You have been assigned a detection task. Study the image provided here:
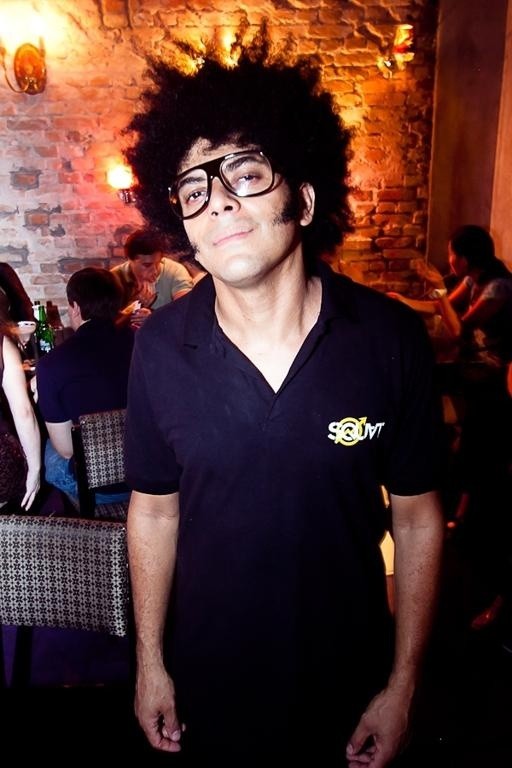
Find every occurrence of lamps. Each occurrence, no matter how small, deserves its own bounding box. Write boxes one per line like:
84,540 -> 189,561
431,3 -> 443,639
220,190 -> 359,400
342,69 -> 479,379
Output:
1,9 -> 50,96
107,165 -> 140,208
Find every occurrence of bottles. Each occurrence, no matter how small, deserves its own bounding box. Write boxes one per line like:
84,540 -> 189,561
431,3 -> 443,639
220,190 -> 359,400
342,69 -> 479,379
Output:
32,300 -> 59,356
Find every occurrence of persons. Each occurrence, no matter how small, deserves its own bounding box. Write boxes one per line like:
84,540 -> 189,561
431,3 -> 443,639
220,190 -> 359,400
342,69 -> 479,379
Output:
382,220 -> 511,368
107,228 -> 195,330
0,260 -> 36,323
29,267 -> 137,507
114,21 -> 449,767
0,328 -> 43,514
429,360 -> 511,631
384,257 -> 463,343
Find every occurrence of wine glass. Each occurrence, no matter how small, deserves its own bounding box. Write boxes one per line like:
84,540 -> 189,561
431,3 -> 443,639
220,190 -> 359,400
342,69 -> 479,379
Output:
13,325 -> 36,372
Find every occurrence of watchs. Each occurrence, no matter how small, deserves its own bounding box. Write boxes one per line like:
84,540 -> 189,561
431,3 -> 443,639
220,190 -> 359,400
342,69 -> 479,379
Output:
427,288 -> 448,301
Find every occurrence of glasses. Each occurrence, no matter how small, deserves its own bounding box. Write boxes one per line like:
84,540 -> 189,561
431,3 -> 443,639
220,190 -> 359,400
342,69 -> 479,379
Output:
159,148 -> 278,222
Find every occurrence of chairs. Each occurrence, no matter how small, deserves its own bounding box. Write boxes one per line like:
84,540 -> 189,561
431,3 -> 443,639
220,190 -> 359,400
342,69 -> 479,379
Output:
0,512 -> 131,768
72,409 -> 135,519
